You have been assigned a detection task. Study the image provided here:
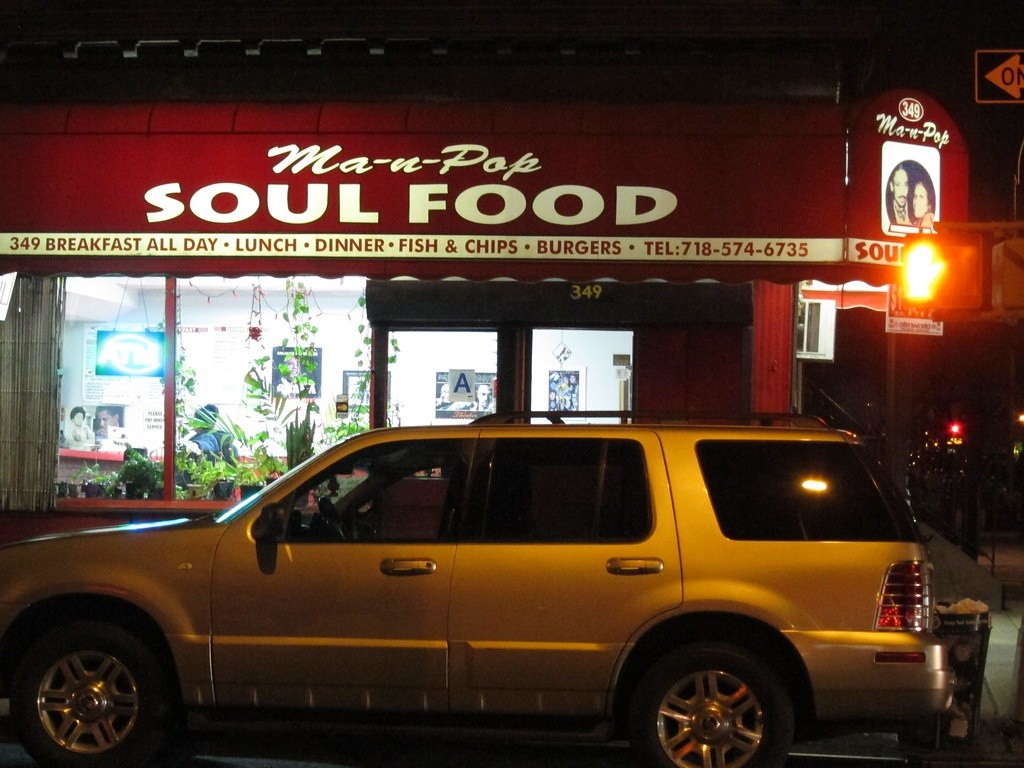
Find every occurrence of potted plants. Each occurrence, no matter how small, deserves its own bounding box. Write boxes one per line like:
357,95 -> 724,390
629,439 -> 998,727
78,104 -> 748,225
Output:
69,284 -> 401,502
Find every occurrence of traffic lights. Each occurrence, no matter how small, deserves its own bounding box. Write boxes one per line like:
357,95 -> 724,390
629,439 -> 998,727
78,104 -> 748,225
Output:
902,233 -> 986,309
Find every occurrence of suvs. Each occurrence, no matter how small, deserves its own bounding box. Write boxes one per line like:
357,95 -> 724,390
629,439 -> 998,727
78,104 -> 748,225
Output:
0,410 -> 956,768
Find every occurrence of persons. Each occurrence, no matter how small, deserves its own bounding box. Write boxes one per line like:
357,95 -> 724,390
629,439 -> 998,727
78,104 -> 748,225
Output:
64,406 -> 124,451
888,166 -> 934,227
436,382 -> 494,412
180,404 -> 240,463
278,352 -> 317,395
549,370 -> 579,411
